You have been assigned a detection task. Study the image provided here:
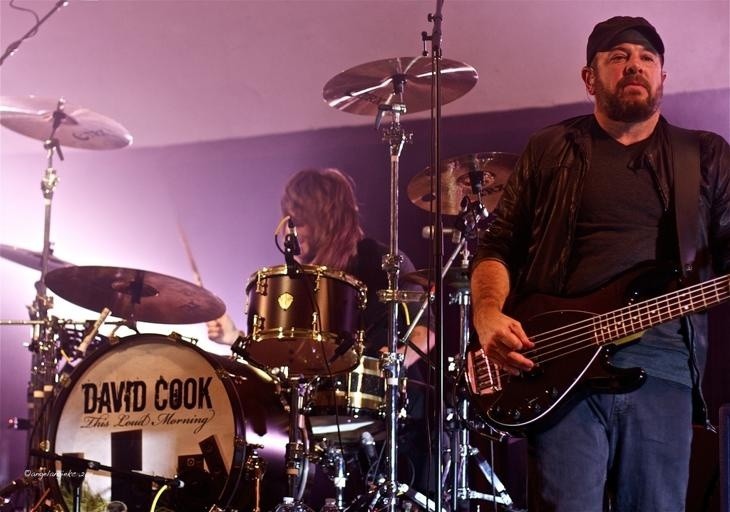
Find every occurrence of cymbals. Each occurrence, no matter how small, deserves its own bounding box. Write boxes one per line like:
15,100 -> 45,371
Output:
407,151 -> 520,215
322,56 -> 479,116
44,266 -> 226,324
0,95 -> 134,151
0,243 -> 77,273
401,264 -> 473,290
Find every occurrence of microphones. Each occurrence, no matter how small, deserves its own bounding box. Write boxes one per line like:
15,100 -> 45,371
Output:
423,226 -> 457,240
288,219 -> 301,256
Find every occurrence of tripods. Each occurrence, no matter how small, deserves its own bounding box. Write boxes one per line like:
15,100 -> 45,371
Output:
441,291 -> 513,512
341,104 -> 447,512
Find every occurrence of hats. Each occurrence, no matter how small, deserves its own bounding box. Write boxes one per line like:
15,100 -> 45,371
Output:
586,17 -> 665,67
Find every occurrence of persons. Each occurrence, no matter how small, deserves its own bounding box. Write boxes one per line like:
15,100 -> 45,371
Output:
467,14 -> 729,511
203,164 -> 438,369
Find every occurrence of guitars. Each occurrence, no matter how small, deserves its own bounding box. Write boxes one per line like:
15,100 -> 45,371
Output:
467,273 -> 730,439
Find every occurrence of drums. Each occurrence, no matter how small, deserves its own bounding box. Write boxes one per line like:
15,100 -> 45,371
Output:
245,263 -> 368,377
44,333 -> 316,512
309,348 -> 411,446
